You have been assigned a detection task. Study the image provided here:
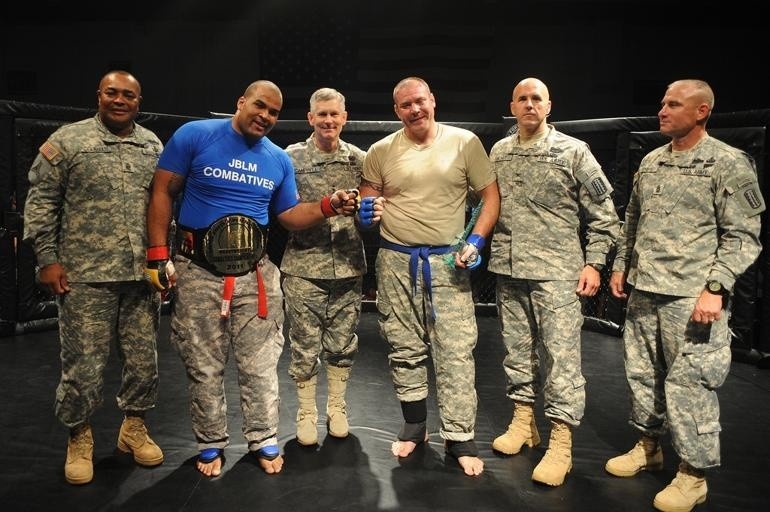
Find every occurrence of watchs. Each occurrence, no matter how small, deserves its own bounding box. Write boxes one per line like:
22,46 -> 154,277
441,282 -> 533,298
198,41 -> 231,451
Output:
704,280 -> 730,299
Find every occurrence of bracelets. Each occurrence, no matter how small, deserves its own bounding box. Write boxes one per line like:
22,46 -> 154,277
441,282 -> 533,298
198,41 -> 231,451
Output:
589,262 -> 604,272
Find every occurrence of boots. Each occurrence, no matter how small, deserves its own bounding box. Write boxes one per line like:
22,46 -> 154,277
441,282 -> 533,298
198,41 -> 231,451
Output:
654,464 -> 707,512
117,411 -> 164,467
604,437 -> 664,476
296,375 -> 318,447
64,423 -> 94,484
493,400 -> 541,457
530,418 -> 572,488
326,366 -> 349,439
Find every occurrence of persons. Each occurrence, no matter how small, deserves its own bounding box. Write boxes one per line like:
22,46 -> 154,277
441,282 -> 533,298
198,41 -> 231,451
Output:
605,79 -> 766,512
354,74 -> 502,475
277,86 -> 369,449
146,79 -> 361,477
24,70 -> 167,485
489,76 -> 620,486
